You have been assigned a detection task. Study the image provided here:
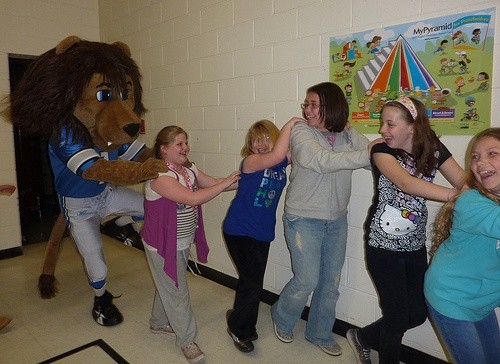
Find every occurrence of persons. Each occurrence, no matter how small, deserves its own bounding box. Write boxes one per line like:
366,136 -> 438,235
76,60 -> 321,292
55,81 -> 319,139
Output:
346,97 -> 470,364
0,184 -> 16,331
270,82 -> 387,356
422,127 -> 500,364
222,117 -> 351,353
139,125 -> 241,364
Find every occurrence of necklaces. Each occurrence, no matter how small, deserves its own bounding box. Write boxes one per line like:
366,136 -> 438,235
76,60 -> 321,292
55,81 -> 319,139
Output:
167,165 -> 194,192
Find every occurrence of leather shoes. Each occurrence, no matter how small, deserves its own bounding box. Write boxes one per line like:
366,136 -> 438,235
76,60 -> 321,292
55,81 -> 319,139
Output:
226,309 -> 258,352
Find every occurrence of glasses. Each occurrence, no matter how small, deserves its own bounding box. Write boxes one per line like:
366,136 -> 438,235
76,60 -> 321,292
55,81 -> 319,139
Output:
253,136 -> 270,143
301,103 -> 321,109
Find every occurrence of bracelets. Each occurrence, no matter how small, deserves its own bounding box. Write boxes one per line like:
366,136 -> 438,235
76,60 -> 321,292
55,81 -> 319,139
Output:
447,188 -> 455,202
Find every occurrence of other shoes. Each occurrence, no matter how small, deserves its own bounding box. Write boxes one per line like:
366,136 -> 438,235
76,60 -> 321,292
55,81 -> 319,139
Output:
0,316 -> 13,330
108,217 -> 141,245
91,292 -> 123,327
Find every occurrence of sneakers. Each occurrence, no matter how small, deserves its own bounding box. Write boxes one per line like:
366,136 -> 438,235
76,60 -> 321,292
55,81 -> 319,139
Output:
305,331 -> 342,356
150,323 -> 176,336
180,342 -> 205,363
273,320 -> 294,343
346,328 -> 372,364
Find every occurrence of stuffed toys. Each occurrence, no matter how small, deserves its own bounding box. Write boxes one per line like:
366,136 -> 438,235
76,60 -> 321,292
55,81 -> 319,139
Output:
0,35 -> 192,327
